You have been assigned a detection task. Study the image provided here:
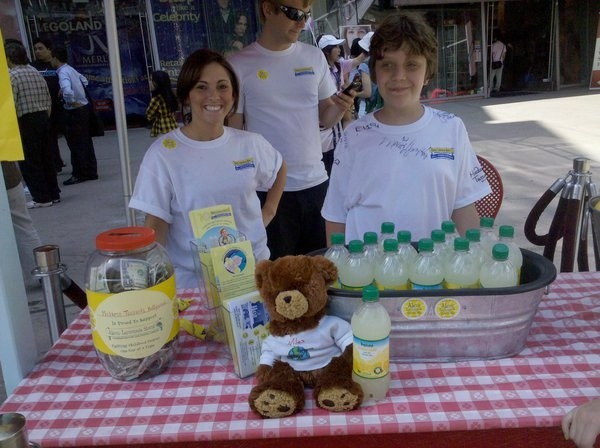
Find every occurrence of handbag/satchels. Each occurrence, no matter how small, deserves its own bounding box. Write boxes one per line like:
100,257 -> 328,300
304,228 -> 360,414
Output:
491,43 -> 504,70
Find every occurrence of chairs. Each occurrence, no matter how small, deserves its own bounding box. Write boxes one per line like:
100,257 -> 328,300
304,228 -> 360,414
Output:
472,155 -> 504,221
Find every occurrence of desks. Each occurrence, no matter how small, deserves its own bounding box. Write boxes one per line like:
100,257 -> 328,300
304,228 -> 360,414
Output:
0,270 -> 600,447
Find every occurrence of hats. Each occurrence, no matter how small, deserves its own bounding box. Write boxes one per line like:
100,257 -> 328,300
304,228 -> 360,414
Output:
357,31 -> 375,53
318,34 -> 346,50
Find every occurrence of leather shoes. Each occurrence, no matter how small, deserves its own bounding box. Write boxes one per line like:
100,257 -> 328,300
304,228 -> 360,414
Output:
81,175 -> 98,180
63,176 -> 85,185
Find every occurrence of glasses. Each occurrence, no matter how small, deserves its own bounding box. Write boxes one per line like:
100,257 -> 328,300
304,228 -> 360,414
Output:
279,3 -> 312,22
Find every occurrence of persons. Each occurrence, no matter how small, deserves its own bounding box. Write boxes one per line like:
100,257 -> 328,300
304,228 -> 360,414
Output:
318,119 -> 342,177
146,71 -> 180,138
50,47 -> 98,186
29,42 -> 68,175
0,28 -> 43,288
321,13 -> 492,248
467,32 -> 490,96
350,37 -> 363,84
224,9 -> 246,51
313,34 -> 369,95
562,397 -> 600,448
347,31 -> 384,119
2,38 -> 60,208
222,0 -> 361,262
216,0 -> 234,34
486,36 -> 507,92
128,49 -> 287,290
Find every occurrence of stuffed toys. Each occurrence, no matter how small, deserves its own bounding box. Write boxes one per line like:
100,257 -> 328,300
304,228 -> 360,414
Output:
249,253 -> 364,420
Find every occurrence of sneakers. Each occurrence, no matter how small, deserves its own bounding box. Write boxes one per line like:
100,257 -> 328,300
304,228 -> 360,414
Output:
26,200 -> 52,208
52,198 -> 60,202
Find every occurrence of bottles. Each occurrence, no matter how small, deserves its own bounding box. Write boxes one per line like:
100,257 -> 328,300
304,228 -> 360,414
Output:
446,237 -> 479,289
431,229 -> 448,260
350,285 -> 391,402
324,233 -> 349,290
442,221 -> 459,249
497,225 -> 523,284
364,232 -> 381,262
480,244 -> 517,288
83,226 -> 180,382
465,229 -> 487,261
397,230 -> 419,263
479,218 -> 499,248
409,238 -> 444,290
375,239 -> 409,290
378,222 -> 399,246
339,240 -> 375,291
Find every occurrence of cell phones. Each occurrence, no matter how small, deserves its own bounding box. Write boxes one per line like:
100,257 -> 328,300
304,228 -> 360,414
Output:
340,72 -> 362,96
